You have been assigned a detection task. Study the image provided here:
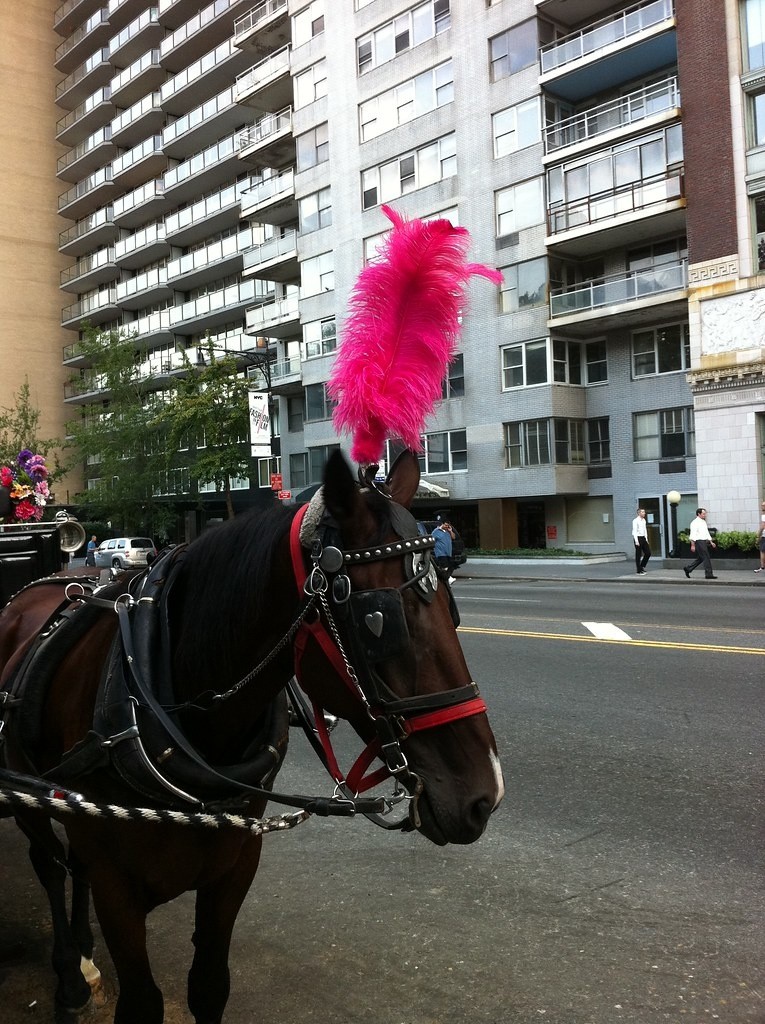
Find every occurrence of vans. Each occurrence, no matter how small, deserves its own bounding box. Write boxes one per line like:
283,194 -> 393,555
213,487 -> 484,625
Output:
84,537 -> 158,570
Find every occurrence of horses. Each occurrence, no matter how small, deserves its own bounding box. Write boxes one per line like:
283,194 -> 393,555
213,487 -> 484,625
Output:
0,443 -> 504,1024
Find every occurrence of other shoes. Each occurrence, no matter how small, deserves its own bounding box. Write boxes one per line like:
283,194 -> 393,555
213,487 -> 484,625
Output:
705,575 -> 717,579
641,566 -> 648,574
683,567 -> 690,578
448,576 -> 456,585
636,571 -> 645,575
754,568 -> 765,573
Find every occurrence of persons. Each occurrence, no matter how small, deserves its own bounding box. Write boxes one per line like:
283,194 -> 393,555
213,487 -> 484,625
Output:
754,502 -> 765,573
632,508 -> 651,575
87,535 -> 99,567
682,508 -> 718,579
431,518 -> 457,585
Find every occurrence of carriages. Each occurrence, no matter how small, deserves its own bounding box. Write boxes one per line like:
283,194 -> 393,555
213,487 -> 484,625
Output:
0,444 -> 506,1024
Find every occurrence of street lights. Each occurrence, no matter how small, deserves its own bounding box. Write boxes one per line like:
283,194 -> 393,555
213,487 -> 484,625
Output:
666,490 -> 682,558
194,346 -> 277,474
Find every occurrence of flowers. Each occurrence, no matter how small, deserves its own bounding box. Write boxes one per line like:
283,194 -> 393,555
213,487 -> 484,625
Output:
0,445 -> 52,522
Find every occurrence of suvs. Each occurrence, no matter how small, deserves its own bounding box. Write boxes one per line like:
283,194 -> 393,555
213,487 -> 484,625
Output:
415,519 -> 467,570
